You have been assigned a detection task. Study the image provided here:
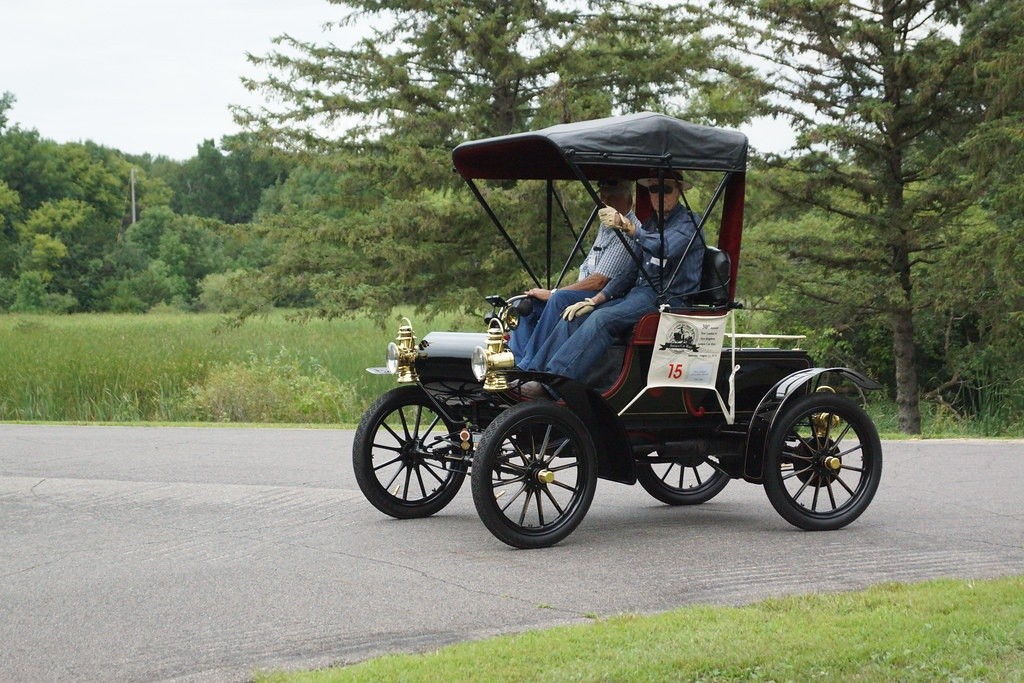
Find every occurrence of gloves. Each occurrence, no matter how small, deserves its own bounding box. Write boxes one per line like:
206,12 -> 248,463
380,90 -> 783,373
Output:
559,297 -> 596,321
597,201 -> 635,236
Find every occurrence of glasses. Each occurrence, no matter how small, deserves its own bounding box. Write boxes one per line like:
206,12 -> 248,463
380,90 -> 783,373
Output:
649,184 -> 679,194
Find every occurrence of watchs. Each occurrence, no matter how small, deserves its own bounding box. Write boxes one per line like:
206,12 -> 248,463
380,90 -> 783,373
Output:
623,221 -> 632,232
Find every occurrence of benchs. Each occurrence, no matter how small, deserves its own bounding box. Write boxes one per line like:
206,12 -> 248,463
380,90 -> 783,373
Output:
591,246 -> 731,398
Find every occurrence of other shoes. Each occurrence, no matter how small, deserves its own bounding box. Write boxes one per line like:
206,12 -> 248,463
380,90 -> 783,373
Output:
508,378 -> 558,403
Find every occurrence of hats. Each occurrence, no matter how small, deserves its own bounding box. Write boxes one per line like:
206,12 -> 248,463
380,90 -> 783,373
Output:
595,177 -> 635,193
637,168 -> 693,190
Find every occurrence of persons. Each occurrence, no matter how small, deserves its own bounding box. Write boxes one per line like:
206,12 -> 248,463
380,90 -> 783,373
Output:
508,169 -> 706,402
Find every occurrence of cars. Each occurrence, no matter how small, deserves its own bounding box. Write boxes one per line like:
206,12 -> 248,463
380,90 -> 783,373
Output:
351,111 -> 883,552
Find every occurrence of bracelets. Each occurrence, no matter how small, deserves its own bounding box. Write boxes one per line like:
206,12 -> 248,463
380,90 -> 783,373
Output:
551,289 -> 557,295
